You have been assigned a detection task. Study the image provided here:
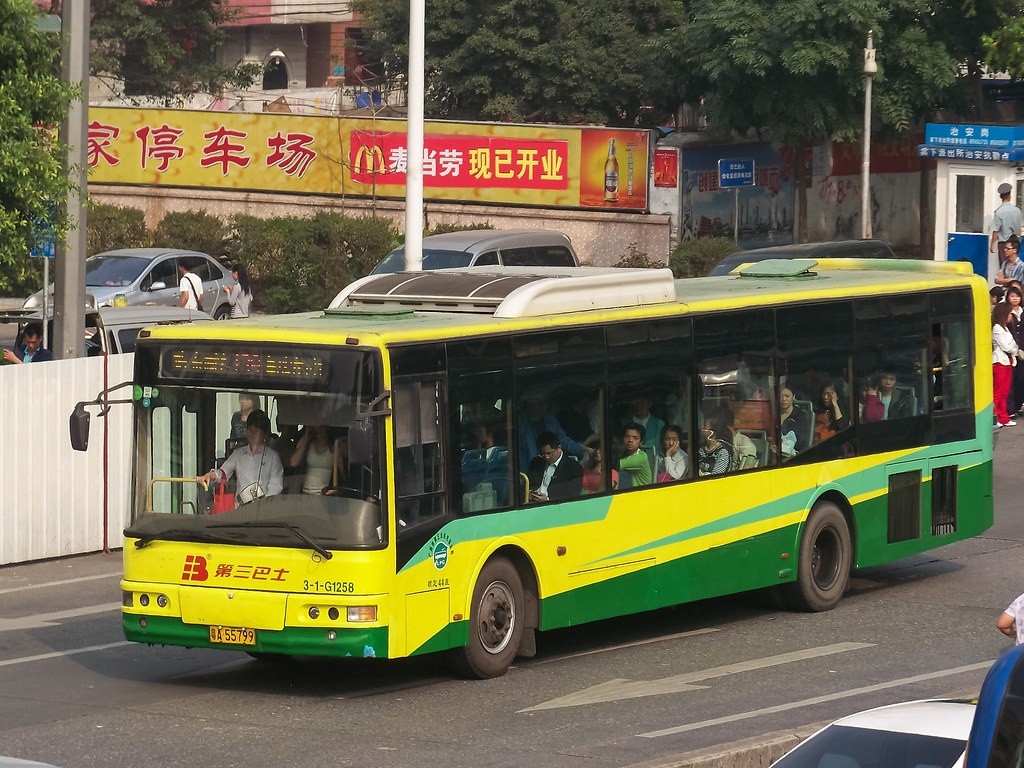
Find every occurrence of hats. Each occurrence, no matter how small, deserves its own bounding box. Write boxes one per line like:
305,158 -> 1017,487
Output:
997,183 -> 1012,194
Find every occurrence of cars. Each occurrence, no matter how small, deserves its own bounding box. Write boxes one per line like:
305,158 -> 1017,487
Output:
21,245 -> 237,318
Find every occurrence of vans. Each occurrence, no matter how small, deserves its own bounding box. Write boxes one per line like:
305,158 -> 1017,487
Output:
703,239 -> 901,276
369,227 -> 581,275
0,308 -> 218,354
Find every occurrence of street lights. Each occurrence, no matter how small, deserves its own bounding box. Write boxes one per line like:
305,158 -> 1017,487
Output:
864,50 -> 877,240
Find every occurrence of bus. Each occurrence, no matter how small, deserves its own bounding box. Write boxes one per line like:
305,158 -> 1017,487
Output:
69,255 -> 995,679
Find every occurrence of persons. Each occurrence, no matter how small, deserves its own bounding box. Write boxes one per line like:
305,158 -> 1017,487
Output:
996,593 -> 1024,646
193,334 -> 948,509
987,184 -> 1024,428
175,259 -> 204,312
2,323 -> 52,364
224,263 -> 253,320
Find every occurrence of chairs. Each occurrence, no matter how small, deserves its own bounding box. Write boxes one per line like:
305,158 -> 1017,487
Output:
793,400 -> 815,454
895,385 -> 917,418
735,429 -> 769,470
817,737 -> 866,768
638,444 -> 659,485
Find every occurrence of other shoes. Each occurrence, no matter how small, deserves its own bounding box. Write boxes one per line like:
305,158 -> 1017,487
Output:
997,422 -> 1003,428
1004,420 -> 1017,426
1009,414 -> 1017,420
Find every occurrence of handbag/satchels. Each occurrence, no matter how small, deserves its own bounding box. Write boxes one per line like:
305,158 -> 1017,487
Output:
197,300 -> 203,311
211,469 -> 236,513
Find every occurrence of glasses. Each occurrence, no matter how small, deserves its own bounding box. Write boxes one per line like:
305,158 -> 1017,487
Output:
538,448 -> 556,460
1004,247 -> 1014,251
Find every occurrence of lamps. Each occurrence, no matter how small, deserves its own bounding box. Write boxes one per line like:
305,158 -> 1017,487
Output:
269,47 -> 286,65
